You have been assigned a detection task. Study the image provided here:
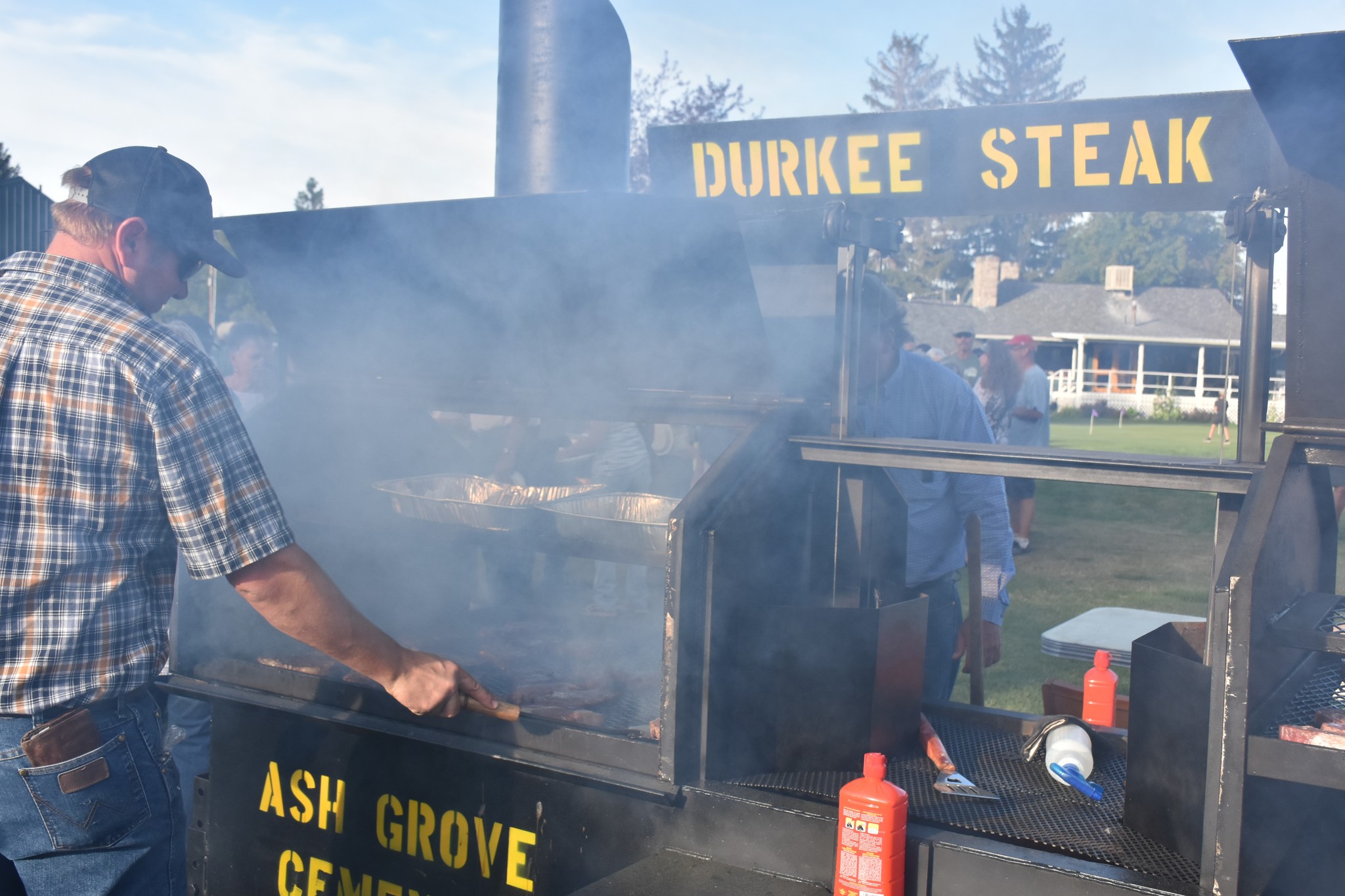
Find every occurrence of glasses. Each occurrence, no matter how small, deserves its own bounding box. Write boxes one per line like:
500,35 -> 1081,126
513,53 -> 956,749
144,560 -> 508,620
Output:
955,332 -> 972,338
166,238 -> 207,283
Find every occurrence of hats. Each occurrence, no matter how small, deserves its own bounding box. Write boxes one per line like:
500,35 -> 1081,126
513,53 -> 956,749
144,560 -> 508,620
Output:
926,347 -> 946,362
952,322 -> 976,336
68,145 -> 250,277
1005,333 -> 1037,352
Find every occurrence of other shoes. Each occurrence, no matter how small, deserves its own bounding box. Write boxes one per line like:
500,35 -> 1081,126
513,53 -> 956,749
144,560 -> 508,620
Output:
1011,540 -> 1031,556
1224,441 -> 1230,445
1203,439 -> 1210,443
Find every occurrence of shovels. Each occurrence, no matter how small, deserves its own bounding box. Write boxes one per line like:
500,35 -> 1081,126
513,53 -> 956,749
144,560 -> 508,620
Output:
919,712 -> 1000,799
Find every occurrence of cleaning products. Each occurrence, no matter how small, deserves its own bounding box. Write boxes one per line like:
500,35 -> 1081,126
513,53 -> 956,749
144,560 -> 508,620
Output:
1041,723 -> 1107,803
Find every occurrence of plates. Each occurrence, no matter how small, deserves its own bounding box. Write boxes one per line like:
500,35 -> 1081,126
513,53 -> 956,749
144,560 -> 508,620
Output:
1039,607 -> 1206,668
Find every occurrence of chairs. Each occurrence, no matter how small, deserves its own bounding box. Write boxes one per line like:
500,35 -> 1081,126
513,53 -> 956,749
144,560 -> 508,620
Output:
1042,677 -> 1129,730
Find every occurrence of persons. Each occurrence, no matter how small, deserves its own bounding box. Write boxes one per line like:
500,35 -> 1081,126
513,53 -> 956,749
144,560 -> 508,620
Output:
159,313 -> 244,418
1004,330 -> 1049,552
0,146 -> 501,895
904,336 -> 945,361
839,276 -> 1016,701
223,321 -> 271,411
938,323 -> 980,388
972,339 -> 1022,446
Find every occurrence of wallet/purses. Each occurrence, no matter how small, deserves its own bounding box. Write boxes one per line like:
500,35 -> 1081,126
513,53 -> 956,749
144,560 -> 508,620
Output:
21,704 -> 101,772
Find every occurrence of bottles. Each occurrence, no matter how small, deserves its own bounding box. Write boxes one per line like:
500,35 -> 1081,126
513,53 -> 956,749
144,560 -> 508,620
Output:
833,752 -> 908,896
1081,649 -> 1118,728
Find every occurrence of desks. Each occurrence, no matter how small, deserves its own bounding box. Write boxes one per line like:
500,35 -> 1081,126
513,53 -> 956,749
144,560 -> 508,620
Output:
1041,607 -> 1207,667
